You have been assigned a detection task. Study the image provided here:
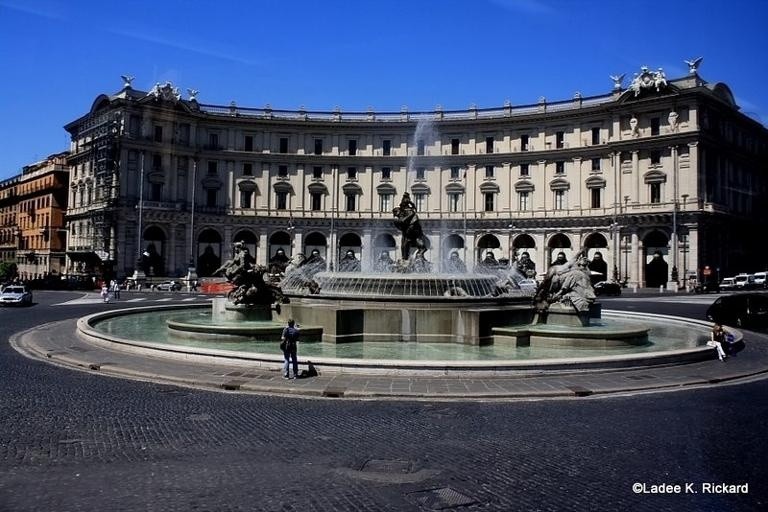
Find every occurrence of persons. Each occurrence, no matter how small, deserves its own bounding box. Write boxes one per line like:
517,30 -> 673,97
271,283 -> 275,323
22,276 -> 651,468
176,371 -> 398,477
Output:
549,269 -> 596,313
391,206 -> 430,262
705,323 -> 727,364
281,319 -> 299,380
226,240 -> 250,276
649,68 -> 667,92
91,275 -> 136,304
631,72 -> 645,97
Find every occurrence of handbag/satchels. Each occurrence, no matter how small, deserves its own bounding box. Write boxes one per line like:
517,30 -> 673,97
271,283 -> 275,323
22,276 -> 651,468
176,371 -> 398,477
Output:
280,341 -> 288,351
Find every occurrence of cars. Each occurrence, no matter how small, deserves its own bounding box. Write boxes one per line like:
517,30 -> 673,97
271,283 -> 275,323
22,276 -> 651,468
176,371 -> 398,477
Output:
157,280 -> 182,291
706,293 -> 767,333
719,271 -> 768,289
518,278 -> 539,290
0,285 -> 33,306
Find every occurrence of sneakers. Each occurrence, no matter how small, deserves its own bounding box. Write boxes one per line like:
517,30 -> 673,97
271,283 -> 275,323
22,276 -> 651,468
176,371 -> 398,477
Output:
282,374 -> 296,380
